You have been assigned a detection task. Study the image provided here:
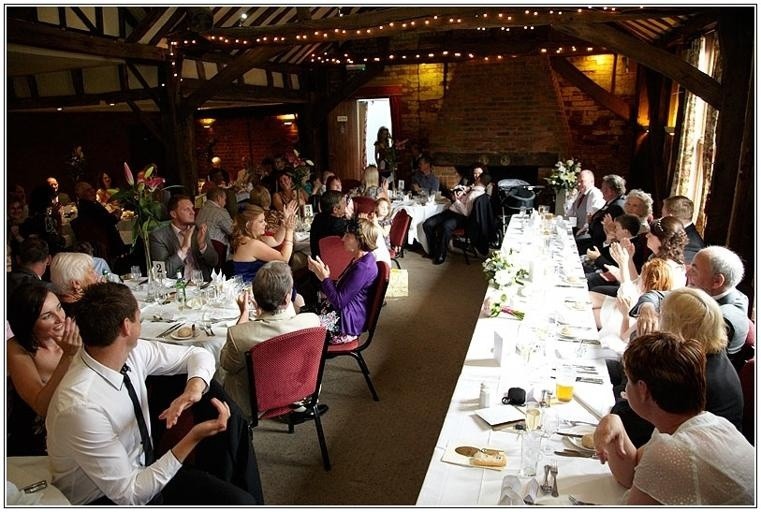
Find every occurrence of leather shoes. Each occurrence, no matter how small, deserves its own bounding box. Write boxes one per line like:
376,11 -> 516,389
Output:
432,253 -> 447,264
292,403 -> 329,424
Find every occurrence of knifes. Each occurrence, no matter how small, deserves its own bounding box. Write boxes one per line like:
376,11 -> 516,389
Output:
154,321 -> 179,337
160,323 -> 185,338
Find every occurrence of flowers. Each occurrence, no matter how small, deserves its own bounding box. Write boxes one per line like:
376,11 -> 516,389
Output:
105,161 -> 186,273
538,156 -> 587,193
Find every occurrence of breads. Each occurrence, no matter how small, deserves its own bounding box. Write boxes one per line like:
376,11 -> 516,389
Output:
582,433 -> 595,448
177,327 -> 191,337
475,451 -> 505,466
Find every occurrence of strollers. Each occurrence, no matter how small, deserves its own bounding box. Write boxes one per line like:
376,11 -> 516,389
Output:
493,178 -> 547,249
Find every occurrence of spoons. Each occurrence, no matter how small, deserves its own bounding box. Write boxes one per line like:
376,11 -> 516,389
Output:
205,323 -> 216,338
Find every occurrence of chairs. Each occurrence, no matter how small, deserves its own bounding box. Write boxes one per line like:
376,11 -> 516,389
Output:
4,174 -> 493,303
324,261 -> 390,402
247,327 -> 332,471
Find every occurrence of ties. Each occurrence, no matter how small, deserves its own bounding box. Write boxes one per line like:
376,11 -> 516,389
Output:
121,372 -> 154,466
179,233 -> 194,266
577,194 -> 586,207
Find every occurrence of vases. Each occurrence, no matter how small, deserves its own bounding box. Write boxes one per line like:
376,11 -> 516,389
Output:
550,187 -> 581,218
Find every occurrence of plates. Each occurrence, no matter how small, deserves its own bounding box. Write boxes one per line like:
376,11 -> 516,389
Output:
169,329 -> 192,340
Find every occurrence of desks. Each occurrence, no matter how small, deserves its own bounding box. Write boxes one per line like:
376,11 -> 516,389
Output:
7,463 -> 71,508
413,213 -> 636,505
118,277 -> 258,388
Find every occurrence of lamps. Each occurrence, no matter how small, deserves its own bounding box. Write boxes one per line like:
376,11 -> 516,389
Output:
198,118 -> 216,129
276,113 -> 297,127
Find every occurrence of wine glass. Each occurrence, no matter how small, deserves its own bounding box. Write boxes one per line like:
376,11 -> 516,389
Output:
94,258 -> 257,325
388,185 -> 449,208
292,215 -> 310,239
467,204 -> 617,505
